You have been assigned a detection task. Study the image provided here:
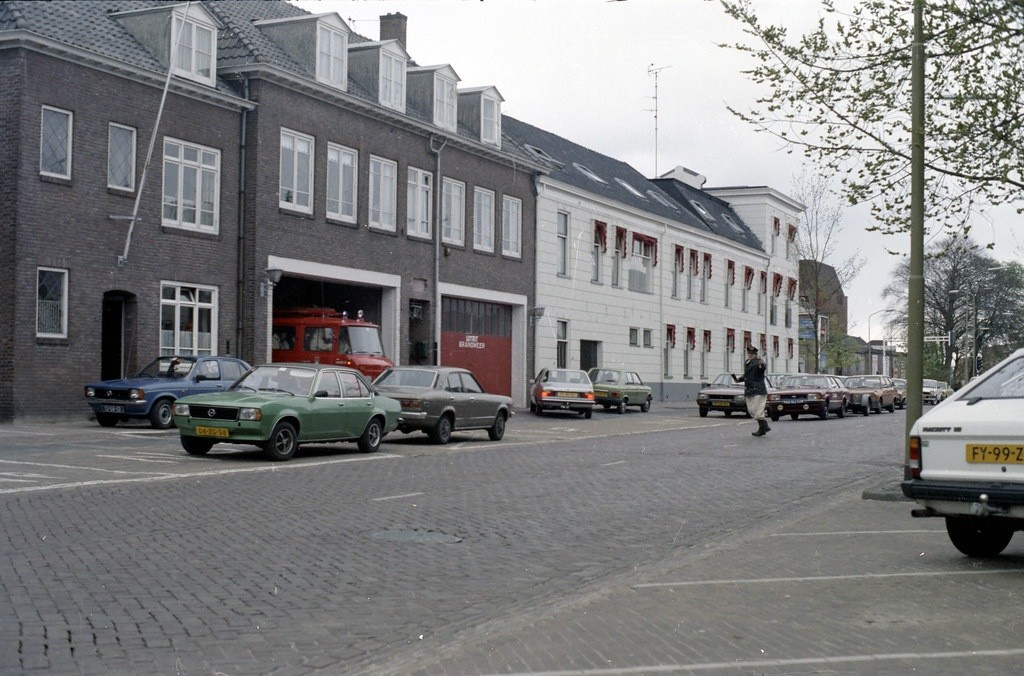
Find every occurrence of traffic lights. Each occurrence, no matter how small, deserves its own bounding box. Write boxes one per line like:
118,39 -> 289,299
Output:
977,356 -> 983,370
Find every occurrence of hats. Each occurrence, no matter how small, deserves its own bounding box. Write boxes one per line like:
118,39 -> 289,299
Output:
746,347 -> 758,355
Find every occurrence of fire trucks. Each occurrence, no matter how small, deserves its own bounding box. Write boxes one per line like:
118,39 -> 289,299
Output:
270,305 -> 396,386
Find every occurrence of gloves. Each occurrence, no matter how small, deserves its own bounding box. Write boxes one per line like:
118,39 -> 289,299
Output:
738,376 -> 744,382
757,363 -> 765,369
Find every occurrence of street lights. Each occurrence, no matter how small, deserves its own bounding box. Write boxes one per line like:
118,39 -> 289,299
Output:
948,289 -> 969,385
869,308 -> 896,374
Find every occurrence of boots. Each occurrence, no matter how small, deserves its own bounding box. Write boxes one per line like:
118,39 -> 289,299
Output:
751,419 -> 771,436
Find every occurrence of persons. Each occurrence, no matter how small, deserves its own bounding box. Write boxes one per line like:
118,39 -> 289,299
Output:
731,347 -> 772,438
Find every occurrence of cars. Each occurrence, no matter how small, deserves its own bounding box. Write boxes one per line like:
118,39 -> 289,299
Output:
765,374 -> 851,421
843,375 -> 898,417
939,381 -> 956,402
172,363 -> 401,463
919,379 -> 942,406
901,347 -> 1024,557
371,365 -> 515,445
889,378 -> 910,410
697,371 -> 773,418
83,354 -> 252,431
587,367 -> 655,414
528,368 -> 596,419
